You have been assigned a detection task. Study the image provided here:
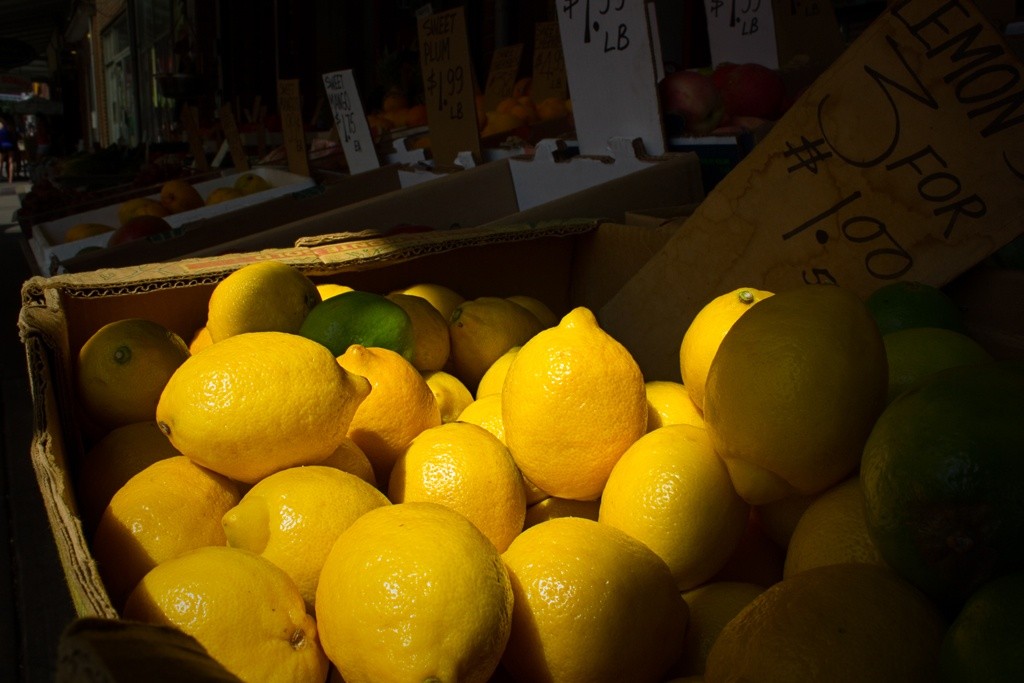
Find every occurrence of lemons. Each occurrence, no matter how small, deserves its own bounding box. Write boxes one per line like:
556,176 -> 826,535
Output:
365,97 -> 571,149
71,260 -> 1024,683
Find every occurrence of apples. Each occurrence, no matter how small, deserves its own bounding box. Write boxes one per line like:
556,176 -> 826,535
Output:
660,62 -> 789,138
66,174 -> 270,242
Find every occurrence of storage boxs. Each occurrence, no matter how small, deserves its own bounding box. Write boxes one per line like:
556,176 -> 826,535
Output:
17,58 -> 1024,683
